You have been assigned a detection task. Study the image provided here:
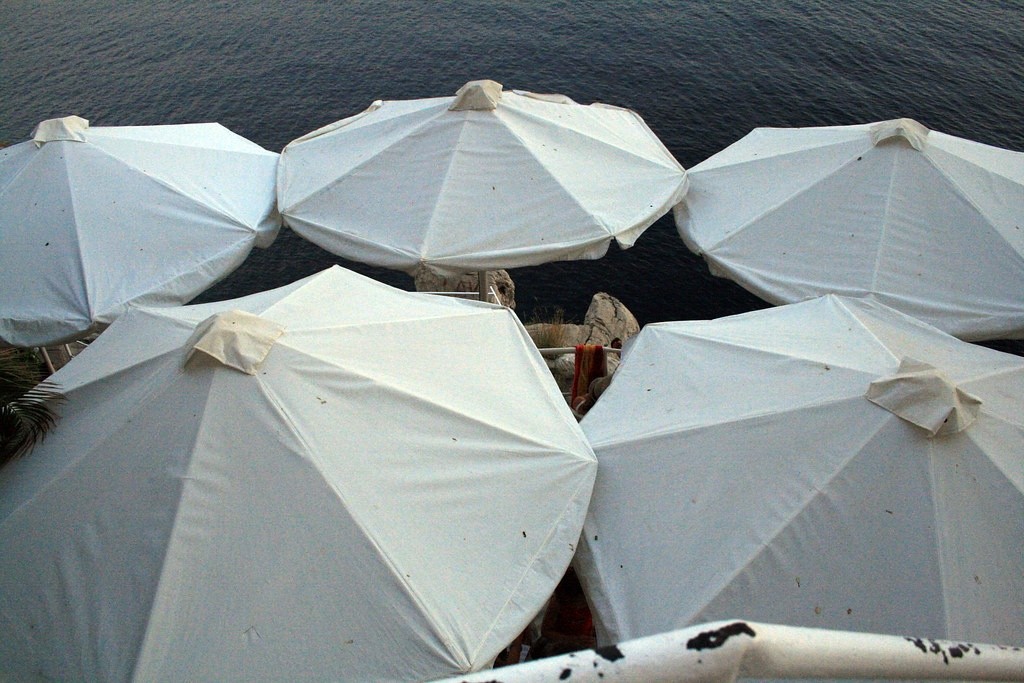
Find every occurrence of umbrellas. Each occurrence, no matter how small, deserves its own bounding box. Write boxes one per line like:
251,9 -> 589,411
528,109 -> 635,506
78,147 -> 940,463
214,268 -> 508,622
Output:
0,81 -> 1024,683
